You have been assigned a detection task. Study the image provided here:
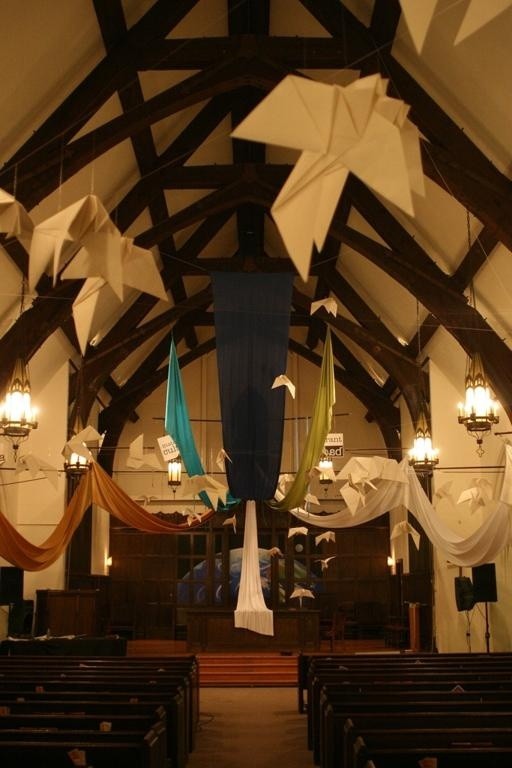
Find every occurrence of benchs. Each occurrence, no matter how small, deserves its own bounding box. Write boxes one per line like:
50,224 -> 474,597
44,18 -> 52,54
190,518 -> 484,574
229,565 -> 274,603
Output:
1,654 -> 201,767
297,653 -> 511,766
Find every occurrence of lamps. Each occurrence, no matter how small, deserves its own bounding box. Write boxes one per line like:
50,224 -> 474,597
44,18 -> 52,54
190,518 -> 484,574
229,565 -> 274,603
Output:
405,137 -> 502,476
62,357 -> 93,479
0,261 -> 39,462
319,452 -> 338,495
167,454 -> 183,494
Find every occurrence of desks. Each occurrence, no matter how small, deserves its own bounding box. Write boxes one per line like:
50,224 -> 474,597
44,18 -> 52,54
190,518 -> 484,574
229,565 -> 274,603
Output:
1,635 -> 127,655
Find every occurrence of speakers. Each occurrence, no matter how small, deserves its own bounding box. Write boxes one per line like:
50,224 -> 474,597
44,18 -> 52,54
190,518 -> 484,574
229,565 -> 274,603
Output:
454,577 -> 475,612
472,563 -> 497,603
0,567 -> 24,606
8,599 -> 34,636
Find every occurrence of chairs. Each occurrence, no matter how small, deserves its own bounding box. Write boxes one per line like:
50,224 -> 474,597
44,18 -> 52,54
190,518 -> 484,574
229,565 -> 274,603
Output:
321,611 -> 346,651
385,602 -> 411,649
